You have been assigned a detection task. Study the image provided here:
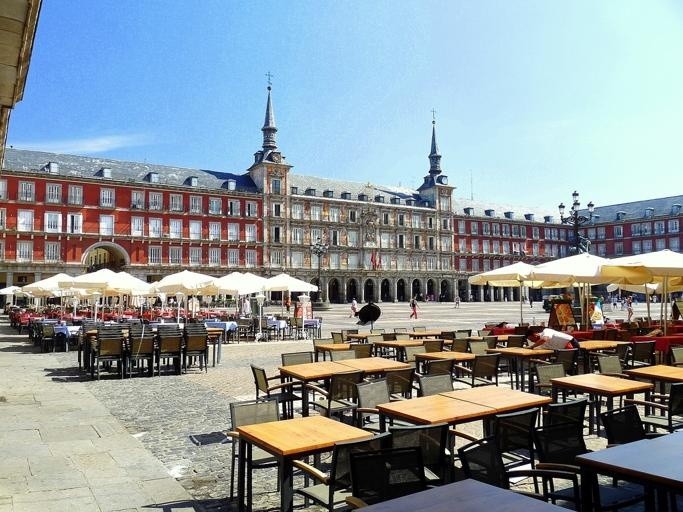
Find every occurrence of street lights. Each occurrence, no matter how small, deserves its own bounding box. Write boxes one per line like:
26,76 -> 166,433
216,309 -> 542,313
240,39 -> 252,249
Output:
557,187 -> 595,322
309,236 -> 330,310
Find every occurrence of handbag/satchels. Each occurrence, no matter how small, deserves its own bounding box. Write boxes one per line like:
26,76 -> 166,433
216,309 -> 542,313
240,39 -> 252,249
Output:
411,299 -> 415,308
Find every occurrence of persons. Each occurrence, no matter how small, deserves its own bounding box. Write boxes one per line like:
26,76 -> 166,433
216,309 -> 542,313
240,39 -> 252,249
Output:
523,326 -> 580,375
407,294 -> 421,320
349,297 -> 360,318
453,294 -> 460,308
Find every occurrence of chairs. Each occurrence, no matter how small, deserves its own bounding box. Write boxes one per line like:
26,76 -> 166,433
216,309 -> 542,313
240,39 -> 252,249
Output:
4,303 -> 228,381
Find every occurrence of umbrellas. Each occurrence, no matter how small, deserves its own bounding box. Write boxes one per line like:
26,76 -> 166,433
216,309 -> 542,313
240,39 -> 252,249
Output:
466,244 -> 683,337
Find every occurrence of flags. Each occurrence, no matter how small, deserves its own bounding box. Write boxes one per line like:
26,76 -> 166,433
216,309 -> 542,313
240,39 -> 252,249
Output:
371,253 -> 376,271
377,255 -> 382,269
